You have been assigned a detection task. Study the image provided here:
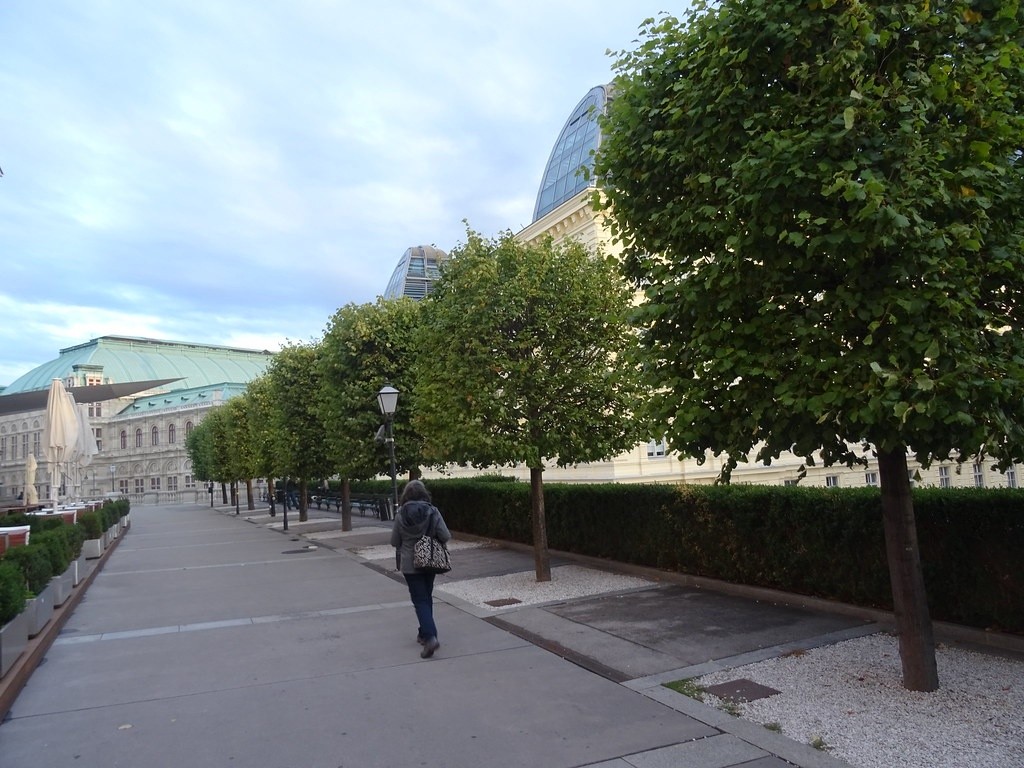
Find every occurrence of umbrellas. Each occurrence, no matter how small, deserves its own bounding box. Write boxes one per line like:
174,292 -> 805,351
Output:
21,377 -> 100,513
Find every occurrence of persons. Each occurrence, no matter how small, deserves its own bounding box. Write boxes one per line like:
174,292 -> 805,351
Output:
390,479 -> 454,660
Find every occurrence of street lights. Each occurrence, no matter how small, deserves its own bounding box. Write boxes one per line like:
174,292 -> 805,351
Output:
109,465 -> 116,493
84,468 -> 96,501
375,385 -> 401,518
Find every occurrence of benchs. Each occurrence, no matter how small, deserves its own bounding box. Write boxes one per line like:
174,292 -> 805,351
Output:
258,492 -> 375,516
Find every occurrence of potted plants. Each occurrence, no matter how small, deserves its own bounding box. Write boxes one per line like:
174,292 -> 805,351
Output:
0,497 -> 131,680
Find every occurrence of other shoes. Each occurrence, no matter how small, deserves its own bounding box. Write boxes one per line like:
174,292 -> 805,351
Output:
421,635 -> 439,658
417,634 -> 427,646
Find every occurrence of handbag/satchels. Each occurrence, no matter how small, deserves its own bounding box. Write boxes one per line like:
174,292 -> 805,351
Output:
413,509 -> 451,574
